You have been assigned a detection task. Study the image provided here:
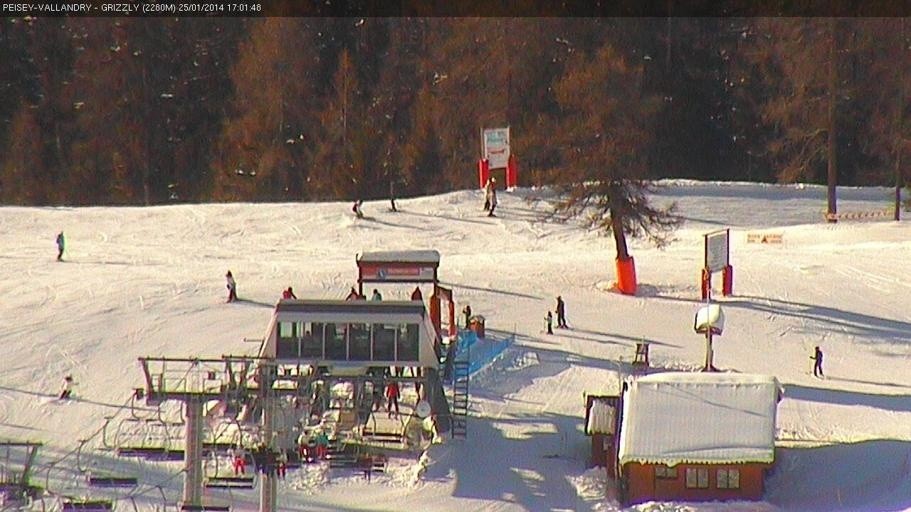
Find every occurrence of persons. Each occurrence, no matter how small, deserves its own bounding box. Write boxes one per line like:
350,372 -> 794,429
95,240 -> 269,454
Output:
810,343 -> 827,381
232,426 -> 374,481
282,286 -> 472,329
59,374 -> 80,400
555,294 -> 567,327
488,185 -> 499,219
56,230 -> 65,260
226,269 -> 237,303
483,176 -> 497,211
386,380 -> 402,420
353,199 -> 364,219
545,311 -> 554,335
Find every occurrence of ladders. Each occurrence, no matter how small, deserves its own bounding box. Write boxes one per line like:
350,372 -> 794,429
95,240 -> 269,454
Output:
451,360 -> 469,440
